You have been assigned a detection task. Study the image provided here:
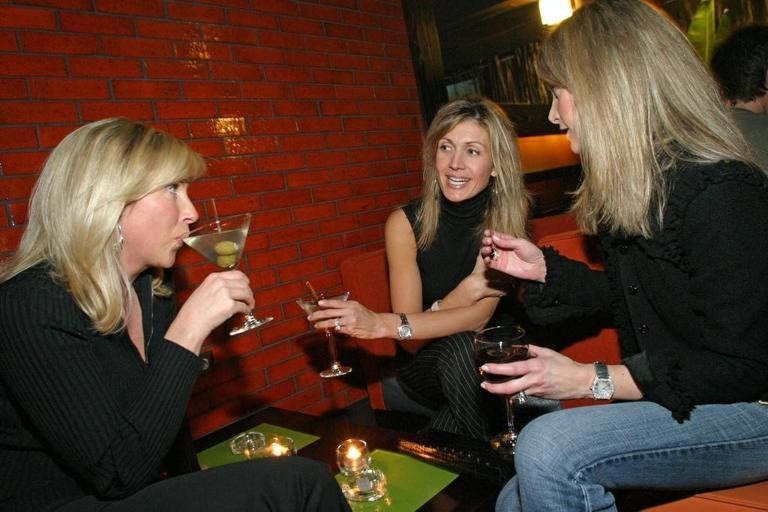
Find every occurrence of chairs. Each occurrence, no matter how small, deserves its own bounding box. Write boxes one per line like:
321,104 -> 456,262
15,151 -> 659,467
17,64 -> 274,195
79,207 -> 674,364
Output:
517,228 -> 768,510
334,210 -> 585,412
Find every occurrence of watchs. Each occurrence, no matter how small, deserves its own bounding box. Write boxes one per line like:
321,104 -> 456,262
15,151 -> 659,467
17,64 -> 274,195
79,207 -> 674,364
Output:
589,362 -> 614,403
397,311 -> 411,342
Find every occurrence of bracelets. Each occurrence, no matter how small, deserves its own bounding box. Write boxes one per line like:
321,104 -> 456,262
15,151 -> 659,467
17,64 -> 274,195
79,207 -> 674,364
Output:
430,298 -> 441,313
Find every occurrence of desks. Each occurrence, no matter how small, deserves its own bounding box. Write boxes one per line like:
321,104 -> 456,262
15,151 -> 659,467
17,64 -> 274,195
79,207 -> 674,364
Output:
191,405 -> 494,512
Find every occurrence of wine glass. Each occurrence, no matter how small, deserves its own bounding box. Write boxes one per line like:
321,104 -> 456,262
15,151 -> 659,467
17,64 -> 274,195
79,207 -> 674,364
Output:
183,210 -> 273,338
472,324 -> 528,456
296,292 -> 352,379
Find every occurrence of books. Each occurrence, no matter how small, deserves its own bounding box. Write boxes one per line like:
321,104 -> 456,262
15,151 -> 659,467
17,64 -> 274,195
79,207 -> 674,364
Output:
439,39 -> 554,107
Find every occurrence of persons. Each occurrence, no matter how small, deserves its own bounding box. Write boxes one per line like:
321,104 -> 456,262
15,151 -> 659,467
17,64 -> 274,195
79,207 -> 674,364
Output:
1,117 -> 350,511
305,95 -> 530,440
479,1 -> 766,511
708,20 -> 768,177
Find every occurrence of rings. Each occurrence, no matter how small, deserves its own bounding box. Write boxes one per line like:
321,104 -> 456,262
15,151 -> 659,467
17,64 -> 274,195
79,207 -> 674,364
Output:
334,317 -> 341,332
517,391 -> 527,407
